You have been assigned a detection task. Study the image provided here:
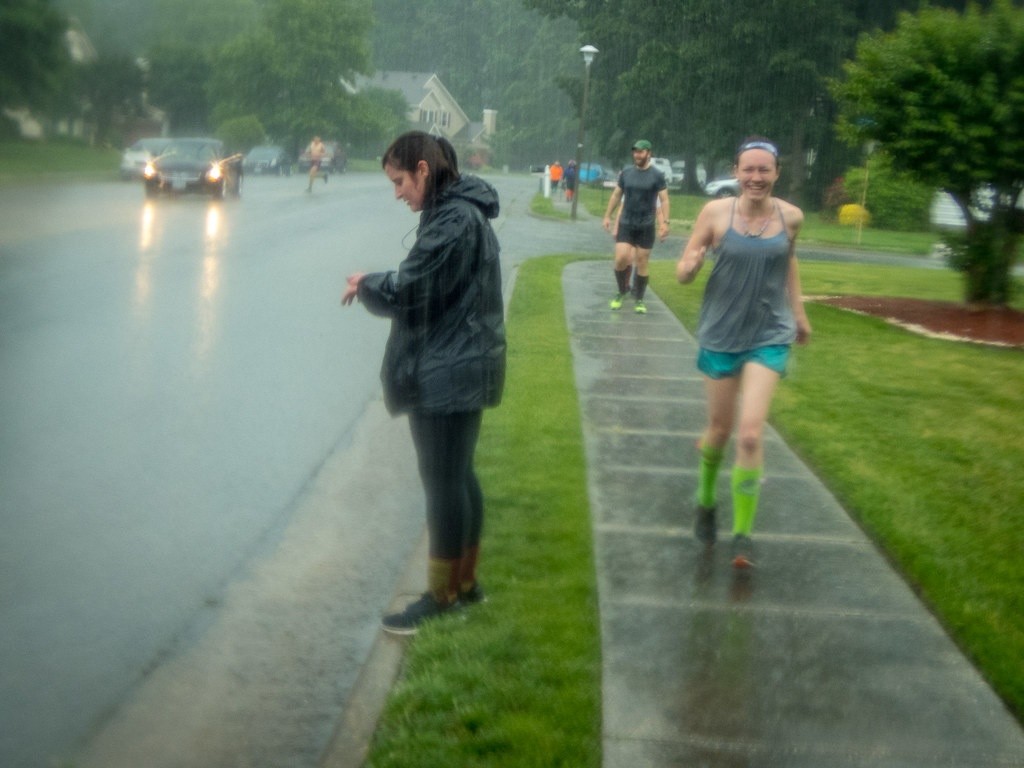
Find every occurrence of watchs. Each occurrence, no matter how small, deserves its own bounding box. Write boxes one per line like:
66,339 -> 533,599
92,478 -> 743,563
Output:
663,219 -> 670,224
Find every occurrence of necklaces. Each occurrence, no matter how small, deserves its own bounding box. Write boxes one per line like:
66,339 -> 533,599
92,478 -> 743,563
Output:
736,197 -> 774,237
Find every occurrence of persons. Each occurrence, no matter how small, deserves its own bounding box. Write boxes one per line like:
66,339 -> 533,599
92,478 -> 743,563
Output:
305,135 -> 328,193
549,160 -> 563,193
603,140 -> 671,315
562,159 -> 576,203
674,135 -> 810,567
341,133 -> 504,636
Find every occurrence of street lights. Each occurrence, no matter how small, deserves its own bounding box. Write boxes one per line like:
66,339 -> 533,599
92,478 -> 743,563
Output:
569,45 -> 600,220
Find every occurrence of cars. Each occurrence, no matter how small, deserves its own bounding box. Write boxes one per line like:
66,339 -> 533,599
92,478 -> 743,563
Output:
241,145 -> 294,177
143,136 -> 243,198
704,179 -> 743,199
650,157 -> 672,184
119,136 -> 172,181
672,160 -> 707,188
298,140 -> 346,175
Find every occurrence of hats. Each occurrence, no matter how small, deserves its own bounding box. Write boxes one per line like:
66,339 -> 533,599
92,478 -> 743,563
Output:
632,140 -> 652,151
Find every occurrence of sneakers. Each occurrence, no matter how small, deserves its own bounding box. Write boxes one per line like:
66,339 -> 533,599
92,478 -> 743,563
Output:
608,290 -> 631,309
694,502 -> 719,547
727,533 -> 754,571
382,595 -> 466,637
634,298 -> 647,315
461,584 -> 485,605
